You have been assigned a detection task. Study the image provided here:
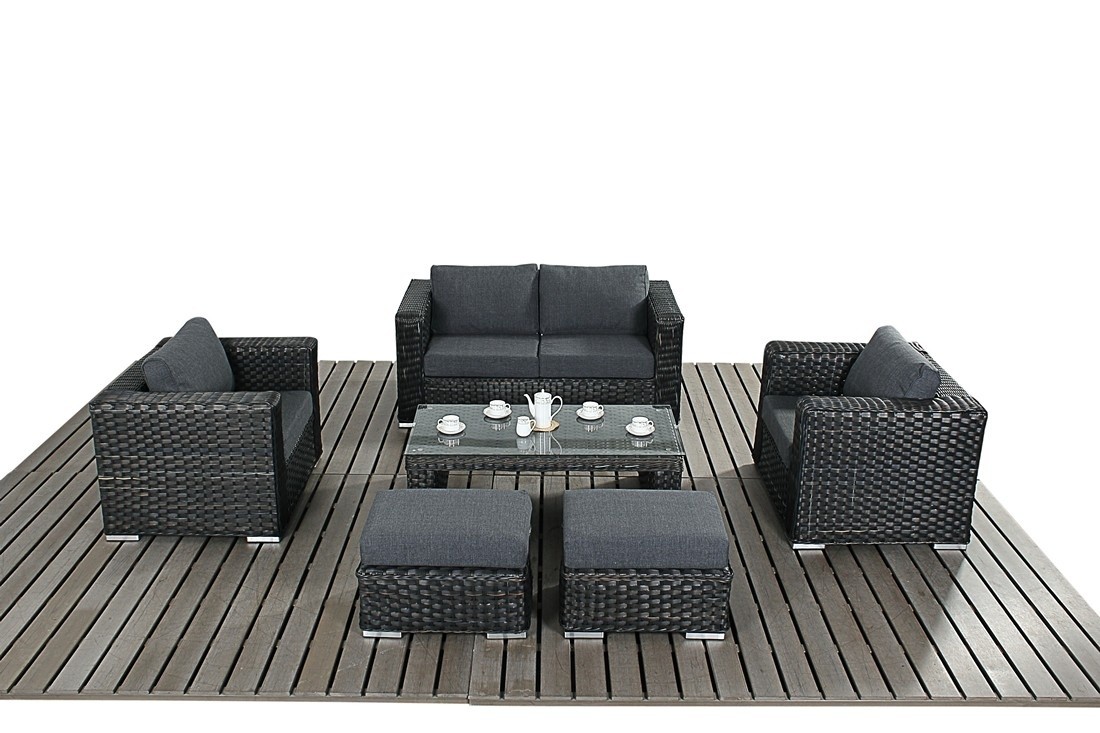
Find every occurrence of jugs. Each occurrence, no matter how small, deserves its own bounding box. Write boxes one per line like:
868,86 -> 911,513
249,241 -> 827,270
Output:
531,431 -> 563,454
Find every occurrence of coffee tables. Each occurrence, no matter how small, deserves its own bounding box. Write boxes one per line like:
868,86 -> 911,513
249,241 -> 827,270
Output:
404,405 -> 682,489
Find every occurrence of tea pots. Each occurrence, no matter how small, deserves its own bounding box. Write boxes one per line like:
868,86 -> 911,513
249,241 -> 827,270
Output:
524,388 -> 563,428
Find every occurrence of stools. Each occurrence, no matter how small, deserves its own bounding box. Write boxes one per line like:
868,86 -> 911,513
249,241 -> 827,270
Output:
562,489 -> 732,641
358,483 -> 530,640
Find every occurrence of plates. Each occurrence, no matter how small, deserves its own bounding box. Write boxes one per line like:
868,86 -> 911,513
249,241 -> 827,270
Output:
483,407 -> 512,418
437,431 -> 466,439
576,408 -> 605,420
484,415 -> 511,423
437,422 -> 466,434
625,423 -> 655,435
576,417 -> 604,424
626,432 -> 655,441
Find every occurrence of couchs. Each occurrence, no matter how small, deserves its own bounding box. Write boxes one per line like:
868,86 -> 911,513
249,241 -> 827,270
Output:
396,263 -> 685,424
756,326 -> 987,549
88,318 -> 322,548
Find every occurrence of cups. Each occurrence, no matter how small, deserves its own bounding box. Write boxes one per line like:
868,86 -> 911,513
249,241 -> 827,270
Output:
584,423 -> 603,432
490,400 -> 510,415
632,416 -> 653,434
438,437 -> 460,448
583,402 -> 603,416
631,439 -> 653,448
516,415 -> 536,438
490,421 -> 511,430
516,438 -> 536,455
438,414 -> 459,432
